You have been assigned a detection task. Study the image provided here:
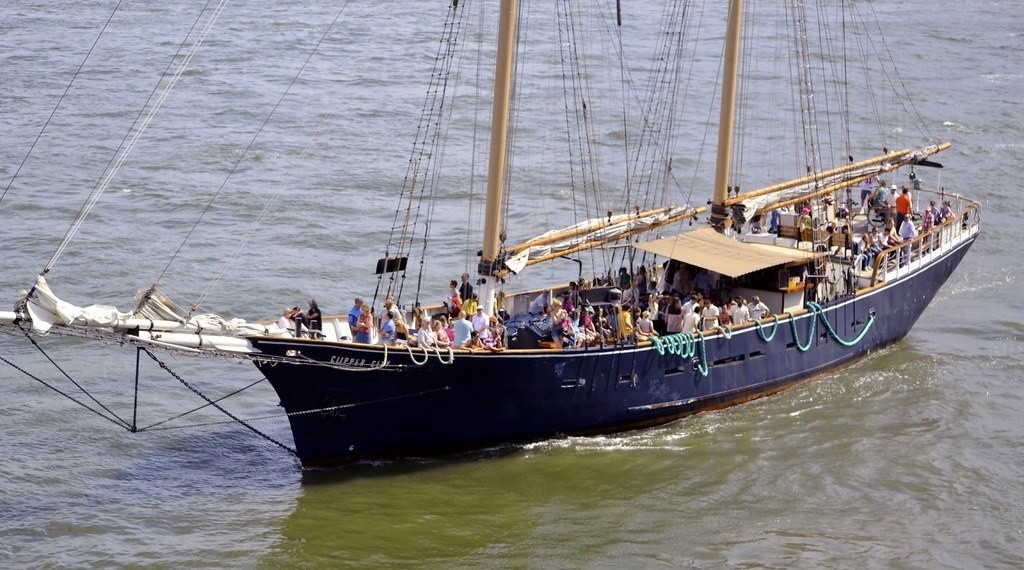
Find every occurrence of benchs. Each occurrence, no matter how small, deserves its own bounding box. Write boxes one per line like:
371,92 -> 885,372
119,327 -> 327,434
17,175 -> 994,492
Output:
534,328 -> 625,348
776,224 -> 854,261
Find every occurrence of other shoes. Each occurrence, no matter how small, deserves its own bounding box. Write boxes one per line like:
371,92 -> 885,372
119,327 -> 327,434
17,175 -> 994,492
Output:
860,210 -> 868,213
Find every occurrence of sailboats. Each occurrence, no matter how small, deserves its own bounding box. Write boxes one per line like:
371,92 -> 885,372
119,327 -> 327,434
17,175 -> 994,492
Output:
0,1 -> 984,478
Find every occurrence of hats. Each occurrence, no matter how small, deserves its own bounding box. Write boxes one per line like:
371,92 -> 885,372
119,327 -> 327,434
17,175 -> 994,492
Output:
891,185 -> 896,189
544,289 -> 550,293
422,317 -> 431,324
554,301 -> 561,305
283,308 -> 293,315
476,304 -> 484,309
801,208 -> 809,213
925,207 -> 930,210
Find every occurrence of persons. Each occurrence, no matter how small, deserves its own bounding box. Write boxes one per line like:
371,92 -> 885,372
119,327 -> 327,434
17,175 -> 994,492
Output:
552,260 -> 771,349
528,290 -> 550,315
349,296 -> 372,345
749,214 -> 764,234
376,272 -> 508,352
770,207 -> 782,234
794,166 -> 957,272
277,299 -> 324,339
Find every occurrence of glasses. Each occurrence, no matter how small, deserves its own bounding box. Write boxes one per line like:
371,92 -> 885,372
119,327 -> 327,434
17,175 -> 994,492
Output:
724,307 -> 727,309
905,216 -> 908,218
462,275 -> 466,278
359,302 -> 363,304
450,283 -> 452,285
